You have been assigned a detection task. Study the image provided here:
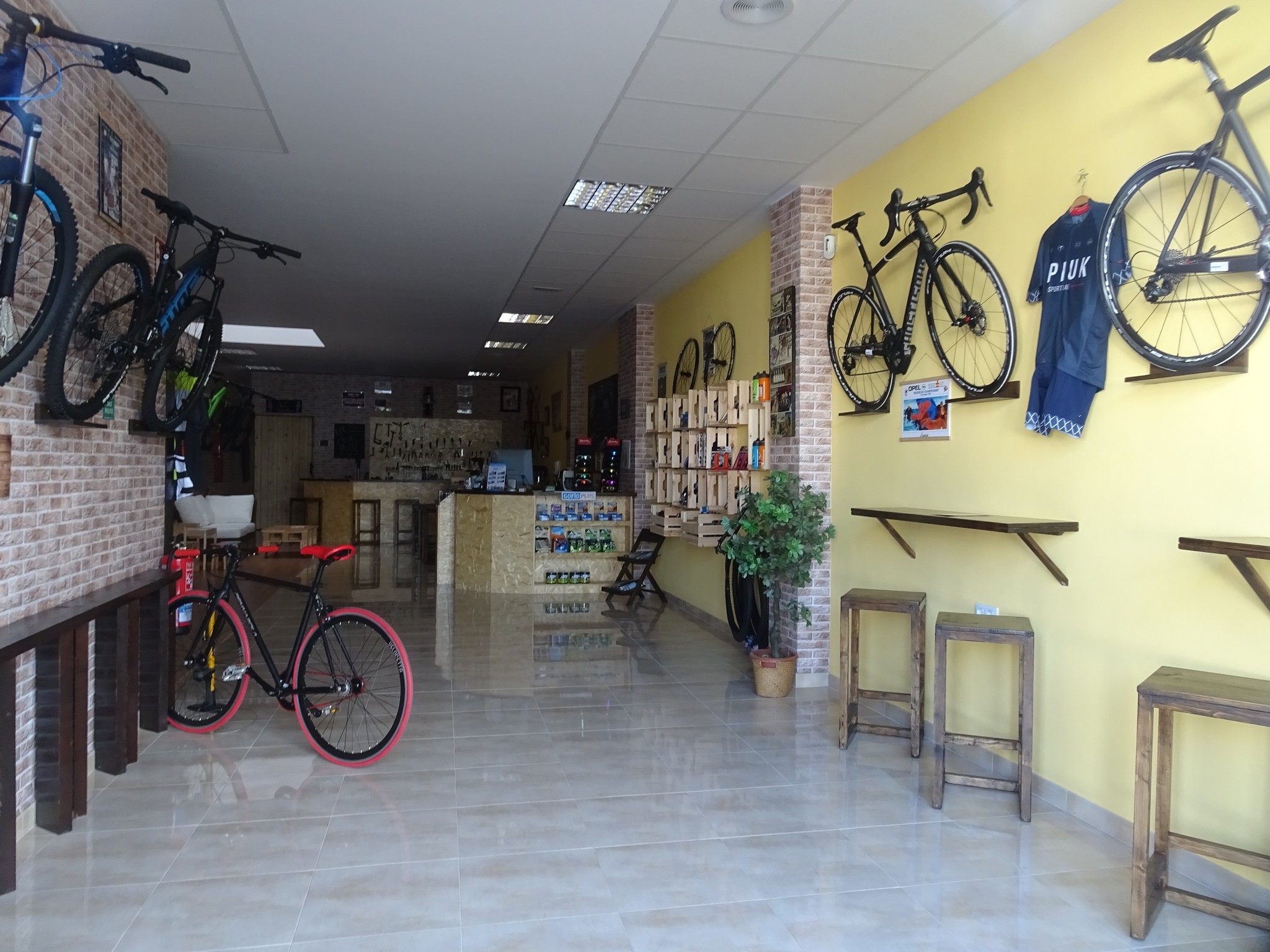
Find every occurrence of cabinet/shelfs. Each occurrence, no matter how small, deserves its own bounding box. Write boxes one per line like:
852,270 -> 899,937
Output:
641,379 -> 771,548
533,495 -> 631,585
601,527 -> 669,607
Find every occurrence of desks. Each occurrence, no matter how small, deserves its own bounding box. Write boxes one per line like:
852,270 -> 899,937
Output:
1176,535 -> 1270,611
261,524 -> 319,560
851,507 -> 1079,587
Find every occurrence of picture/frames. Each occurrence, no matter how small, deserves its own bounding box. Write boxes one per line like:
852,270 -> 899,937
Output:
499,385 -> 522,414
97,113 -> 124,234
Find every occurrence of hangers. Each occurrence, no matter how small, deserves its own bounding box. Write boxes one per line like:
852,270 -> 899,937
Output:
1066,173 -> 1094,216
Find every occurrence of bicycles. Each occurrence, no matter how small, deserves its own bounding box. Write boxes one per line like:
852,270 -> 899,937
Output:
163,544 -> 412,766
1094,4 -> 1270,375
826,168 -> 1017,413
164,370 -> 278,457
47,188 -> 307,437
0,2 -> 195,392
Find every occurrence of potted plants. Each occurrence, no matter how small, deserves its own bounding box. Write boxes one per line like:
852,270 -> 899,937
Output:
720,468 -> 838,700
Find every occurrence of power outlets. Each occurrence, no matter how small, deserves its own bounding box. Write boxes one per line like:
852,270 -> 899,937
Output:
973,602 -> 1000,618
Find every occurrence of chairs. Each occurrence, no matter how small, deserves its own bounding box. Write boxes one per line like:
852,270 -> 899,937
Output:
172,492 -> 257,573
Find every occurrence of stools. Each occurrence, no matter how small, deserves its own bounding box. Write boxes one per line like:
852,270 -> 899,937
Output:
351,498 -> 381,547
837,587 -> 929,759
929,611 -> 1036,823
1129,664 -> 1270,941
393,497 -> 439,564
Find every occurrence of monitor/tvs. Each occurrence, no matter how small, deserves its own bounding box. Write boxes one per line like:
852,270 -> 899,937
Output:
492,449 -> 534,492
